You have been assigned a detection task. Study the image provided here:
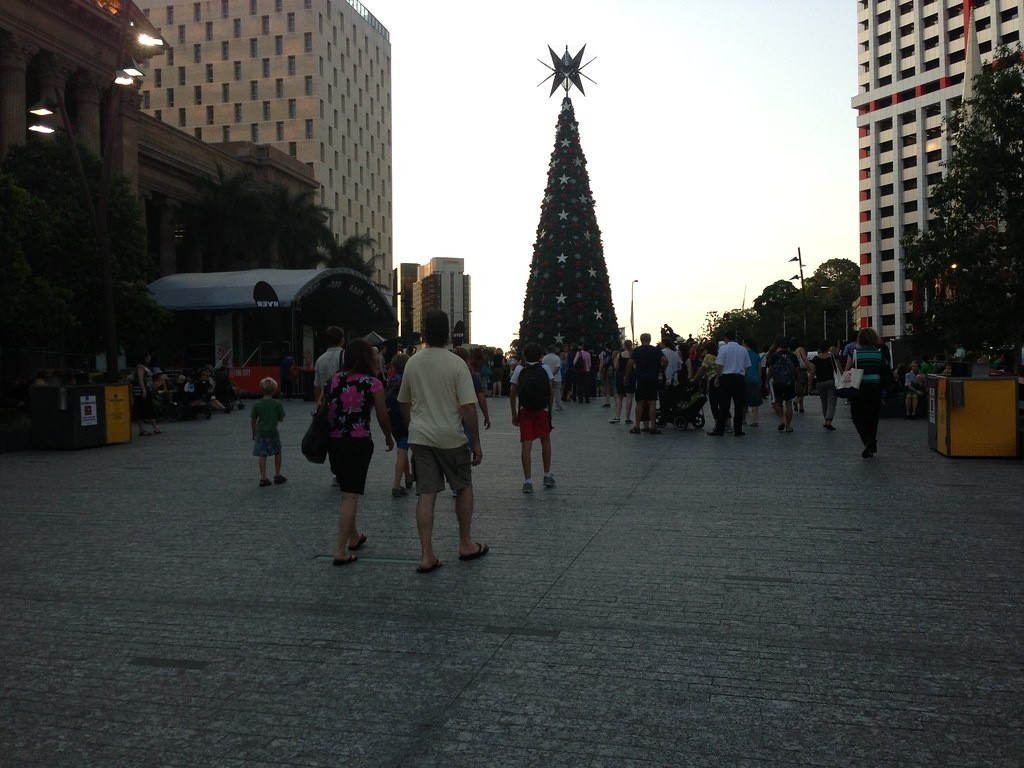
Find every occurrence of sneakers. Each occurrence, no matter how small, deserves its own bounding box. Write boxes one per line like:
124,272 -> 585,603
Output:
522,482 -> 533,492
542,473 -> 555,487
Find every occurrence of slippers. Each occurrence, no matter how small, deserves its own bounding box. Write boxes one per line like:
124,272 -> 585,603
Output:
154,429 -> 162,434
138,431 -> 152,436
415,558 -> 443,574
333,553 -> 357,566
458,542 -> 489,561
349,534 -> 367,551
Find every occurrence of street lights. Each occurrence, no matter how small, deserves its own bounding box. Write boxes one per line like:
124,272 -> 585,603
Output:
630,279 -> 638,347
788,246 -> 806,349
28,1 -> 163,382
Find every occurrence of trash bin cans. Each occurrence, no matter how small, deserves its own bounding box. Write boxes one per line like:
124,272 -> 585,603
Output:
926,359 -> 1018,459
30,367 -> 132,451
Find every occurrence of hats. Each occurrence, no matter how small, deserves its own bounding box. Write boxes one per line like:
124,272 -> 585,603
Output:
151,367 -> 163,376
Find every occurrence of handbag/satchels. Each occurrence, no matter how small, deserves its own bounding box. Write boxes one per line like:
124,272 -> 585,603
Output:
782,371 -> 799,398
575,351 -> 586,375
798,348 -> 864,391
132,380 -> 147,396
301,376 -> 331,464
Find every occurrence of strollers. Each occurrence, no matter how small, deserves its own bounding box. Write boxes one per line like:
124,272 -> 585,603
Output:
655,369 -> 708,432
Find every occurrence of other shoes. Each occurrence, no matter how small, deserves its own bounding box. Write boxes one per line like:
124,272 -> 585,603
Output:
183,404 -> 234,421
391,485 -> 406,497
332,478 -> 340,487
258,478 -> 272,488
561,387 -> 915,459
452,490 -> 456,496
405,473 -> 413,489
273,474 -> 287,485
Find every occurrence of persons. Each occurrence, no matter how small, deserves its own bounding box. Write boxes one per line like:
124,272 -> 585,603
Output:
314,326 -> 345,409
686,334 -> 694,341
661,324 -> 685,349
469,335 -> 890,434
134,353 -> 162,436
384,353 -> 414,496
371,344 -> 417,387
250,377 -> 289,487
452,346 -> 491,498
844,327 -> 893,459
301,339 -> 395,564
80,361 -> 90,385
509,342 -> 556,493
896,361 -> 950,420
278,352 -> 293,400
120,366 -> 240,419
808,341 -> 844,431
396,310 -> 489,573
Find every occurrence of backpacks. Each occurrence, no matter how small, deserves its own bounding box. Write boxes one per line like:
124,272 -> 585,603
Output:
773,351 -> 795,383
516,360 -> 551,411
